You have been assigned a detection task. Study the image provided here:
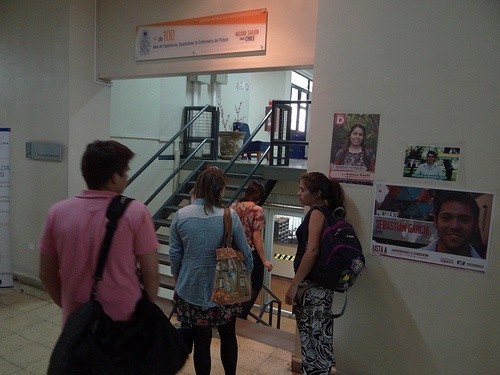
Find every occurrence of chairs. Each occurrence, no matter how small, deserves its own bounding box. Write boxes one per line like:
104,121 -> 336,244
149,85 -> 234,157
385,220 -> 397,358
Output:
233,122 -> 270,162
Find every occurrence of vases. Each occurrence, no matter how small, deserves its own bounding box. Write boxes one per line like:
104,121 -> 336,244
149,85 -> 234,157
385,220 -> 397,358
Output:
218,131 -> 246,156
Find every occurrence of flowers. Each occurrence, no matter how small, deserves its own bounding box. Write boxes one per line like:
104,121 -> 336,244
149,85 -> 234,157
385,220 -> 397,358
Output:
216,96 -> 247,132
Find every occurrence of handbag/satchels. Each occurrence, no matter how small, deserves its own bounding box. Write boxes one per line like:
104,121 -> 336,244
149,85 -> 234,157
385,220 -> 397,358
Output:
169,303 -> 193,354
211,207 -> 250,305
47,294 -> 188,375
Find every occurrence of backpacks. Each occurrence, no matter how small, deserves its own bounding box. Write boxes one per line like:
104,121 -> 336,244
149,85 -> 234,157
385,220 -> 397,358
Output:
308,205 -> 365,292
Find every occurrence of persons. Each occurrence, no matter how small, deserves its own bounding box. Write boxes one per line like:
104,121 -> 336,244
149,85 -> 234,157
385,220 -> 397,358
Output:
230,180 -> 273,320
39,140 -> 159,331
417,190 -> 483,259
285,172 -> 347,375
415,152 -> 444,178
169,169 -> 253,375
334,124 -> 374,171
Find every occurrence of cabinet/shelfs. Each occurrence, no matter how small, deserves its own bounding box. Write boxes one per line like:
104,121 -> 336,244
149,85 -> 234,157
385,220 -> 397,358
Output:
273,217 -> 289,243
